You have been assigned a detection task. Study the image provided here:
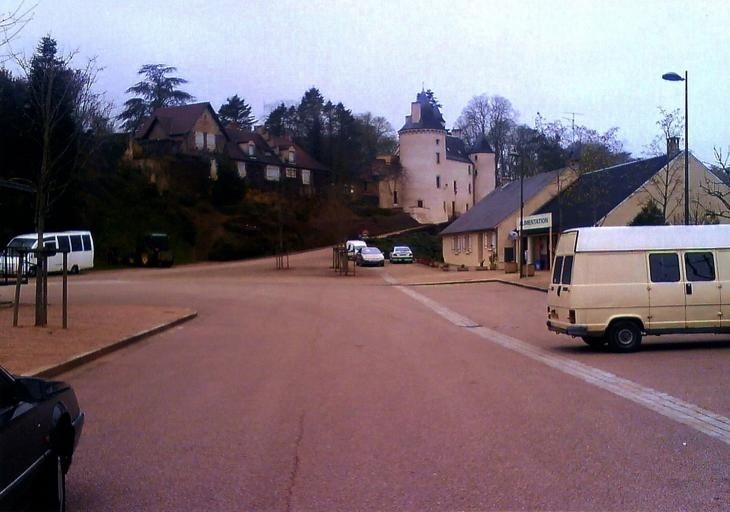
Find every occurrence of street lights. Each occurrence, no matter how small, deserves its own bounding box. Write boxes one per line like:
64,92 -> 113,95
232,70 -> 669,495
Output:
507,143 -> 526,279
660,68 -> 691,226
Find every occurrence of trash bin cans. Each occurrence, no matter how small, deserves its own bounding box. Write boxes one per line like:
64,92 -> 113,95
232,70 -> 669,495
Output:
333,247 -> 348,271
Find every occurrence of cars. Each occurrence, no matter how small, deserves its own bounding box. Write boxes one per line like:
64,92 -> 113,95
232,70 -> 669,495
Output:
0,362 -> 86,511
356,247 -> 386,267
389,245 -> 414,264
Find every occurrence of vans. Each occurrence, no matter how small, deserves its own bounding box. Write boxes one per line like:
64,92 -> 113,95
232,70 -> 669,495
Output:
545,222 -> 730,350
346,239 -> 366,260
1,230 -> 95,278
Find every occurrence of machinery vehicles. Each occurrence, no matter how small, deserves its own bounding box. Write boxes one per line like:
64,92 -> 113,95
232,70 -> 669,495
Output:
108,229 -> 176,268
